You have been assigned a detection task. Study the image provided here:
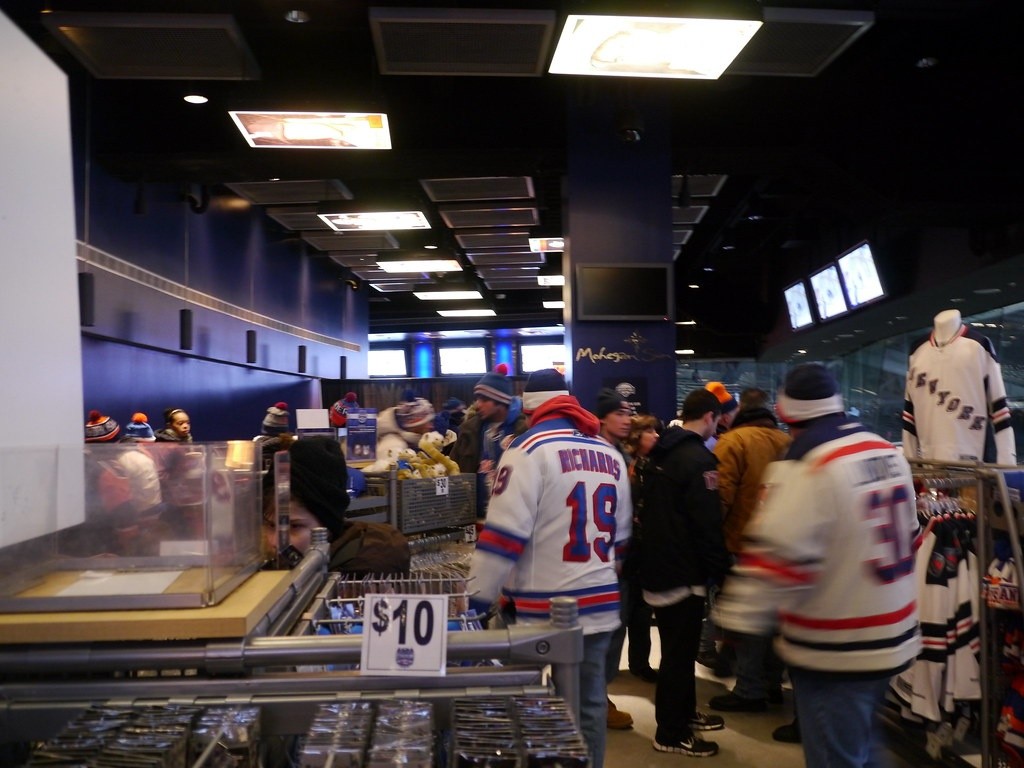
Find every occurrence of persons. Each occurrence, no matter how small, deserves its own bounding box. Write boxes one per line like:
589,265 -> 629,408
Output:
710,363 -> 925,767
900,309 -> 1017,466
375,362 -> 924,768
84,406 -> 206,557
233,401 -> 297,527
631,387 -> 728,759
465,367 -> 632,768
260,435 -> 411,609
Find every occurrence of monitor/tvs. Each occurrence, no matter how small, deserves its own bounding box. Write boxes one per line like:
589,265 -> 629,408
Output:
575,262 -> 673,321
808,262 -> 852,323
438,345 -> 488,376
519,343 -> 567,375
834,238 -> 888,310
782,280 -> 817,332
367,347 -> 408,378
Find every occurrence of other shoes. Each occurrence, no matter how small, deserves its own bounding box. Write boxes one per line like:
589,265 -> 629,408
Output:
630,663 -> 657,683
773,718 -> 802,743
696,649 -> 718,668
709,692 -> 784,711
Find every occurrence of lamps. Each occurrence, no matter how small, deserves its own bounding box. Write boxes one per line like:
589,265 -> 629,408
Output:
183,89 -> 208,104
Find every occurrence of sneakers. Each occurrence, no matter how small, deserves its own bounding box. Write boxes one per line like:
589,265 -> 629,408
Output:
691,712 -> 724,730
654,724 -> 719,756
608,702 -> 633,728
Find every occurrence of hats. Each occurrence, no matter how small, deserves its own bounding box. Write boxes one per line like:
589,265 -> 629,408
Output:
595,388 -> 625,420
523,368 -> 569,412
777,364 -> 845,423
84,409 -> 120,443
271,437 -> 350,532
474,363 -> 513,407
397,390 -> 436,428
262,402 -> 290,435
124,413 -> 156,442
705,381 -> 737,413
329,392 -> 360,426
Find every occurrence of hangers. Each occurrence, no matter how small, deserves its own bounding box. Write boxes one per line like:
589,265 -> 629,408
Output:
913,475 -> 978,523
